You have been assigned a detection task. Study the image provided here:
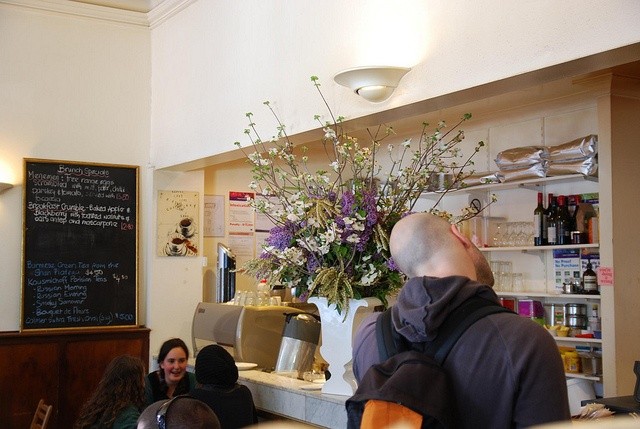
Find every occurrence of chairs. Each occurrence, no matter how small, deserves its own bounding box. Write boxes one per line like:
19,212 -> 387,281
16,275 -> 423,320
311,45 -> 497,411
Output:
30,400 -> 52,429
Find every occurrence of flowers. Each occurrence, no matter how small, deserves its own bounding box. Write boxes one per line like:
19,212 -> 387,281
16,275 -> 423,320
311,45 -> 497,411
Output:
226,73 -> 499,318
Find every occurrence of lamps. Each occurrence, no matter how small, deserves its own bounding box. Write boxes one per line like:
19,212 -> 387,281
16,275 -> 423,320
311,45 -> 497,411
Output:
333,64 -> 411,102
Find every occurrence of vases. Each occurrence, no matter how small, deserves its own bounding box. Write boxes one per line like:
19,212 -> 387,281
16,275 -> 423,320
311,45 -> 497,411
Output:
307,296 -> 384,397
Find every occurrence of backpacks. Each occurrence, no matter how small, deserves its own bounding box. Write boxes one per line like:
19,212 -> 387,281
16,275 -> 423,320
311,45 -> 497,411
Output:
345,299 -> 519,429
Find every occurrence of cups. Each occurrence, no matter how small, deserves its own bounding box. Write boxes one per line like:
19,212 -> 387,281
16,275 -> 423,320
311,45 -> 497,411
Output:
499,272 -> 512,292
491,272 -> 500,292
310,371 -> 326,382
499,262 -> 515,272
489,261 -> 500,272
178,217 -> 192,232
512,272 -> 527,292
301,371 -> 312,382
169,233 -> 186,252
233,289 -> 276,308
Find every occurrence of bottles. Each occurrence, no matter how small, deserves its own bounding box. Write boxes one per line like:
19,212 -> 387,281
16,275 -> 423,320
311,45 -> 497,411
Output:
571,195 -> 581,227
587,303 -> 600,331
533,192 -> 548,246
555,195 -> 572,244
583,255 -> 597,291
546,194 -> 558,245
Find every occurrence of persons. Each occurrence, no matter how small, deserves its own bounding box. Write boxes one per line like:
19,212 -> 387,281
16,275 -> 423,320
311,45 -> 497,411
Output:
351,211 -> 571,429
190,344 -> 259,428
143,337 -> 196,407
75,355 -> 147,429
136,397 -> 221,429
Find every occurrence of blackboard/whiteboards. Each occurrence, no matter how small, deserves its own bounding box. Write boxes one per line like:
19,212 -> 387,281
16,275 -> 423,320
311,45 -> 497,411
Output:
20,158 -> 142,333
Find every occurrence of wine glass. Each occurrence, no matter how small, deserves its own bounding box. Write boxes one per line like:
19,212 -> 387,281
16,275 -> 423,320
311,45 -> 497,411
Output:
516,223 -> 527,247
509,222 -> 517,247
501,222 -> 510,246
526,222 -> 536,246
491,223 -> 504,247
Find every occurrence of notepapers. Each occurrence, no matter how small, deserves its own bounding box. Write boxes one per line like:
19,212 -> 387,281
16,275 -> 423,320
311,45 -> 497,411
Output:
596,266 -> 614,286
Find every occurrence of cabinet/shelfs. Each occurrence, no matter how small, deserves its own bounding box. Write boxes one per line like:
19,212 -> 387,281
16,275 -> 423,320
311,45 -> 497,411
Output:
374,71 -> 640,398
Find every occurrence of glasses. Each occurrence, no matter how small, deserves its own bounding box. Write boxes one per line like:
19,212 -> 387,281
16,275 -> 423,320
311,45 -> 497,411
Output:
154,392 -> 193,427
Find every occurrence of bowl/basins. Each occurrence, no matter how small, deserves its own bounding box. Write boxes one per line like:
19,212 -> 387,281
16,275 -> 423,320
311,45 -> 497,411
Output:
565,315 -> 587,329
564,303 -> 587,316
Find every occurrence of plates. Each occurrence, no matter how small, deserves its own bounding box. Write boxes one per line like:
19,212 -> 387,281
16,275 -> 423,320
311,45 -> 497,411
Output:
174,227 -> 195,238
162,245 -> 189,256
299,384 -> 322,390
235,362 -> 258,371
